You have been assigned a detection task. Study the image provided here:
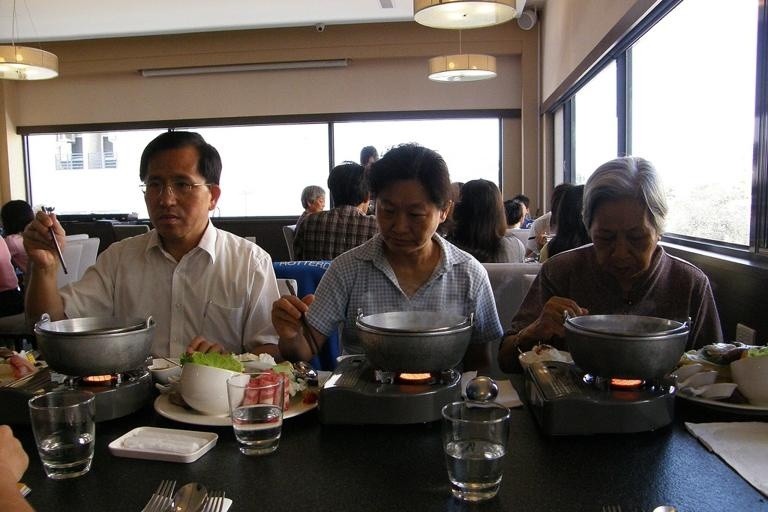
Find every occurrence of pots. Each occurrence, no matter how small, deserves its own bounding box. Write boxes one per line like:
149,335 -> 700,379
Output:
33,311 -> 157,376
562,309 -> 691,382
355,306 -> 476,375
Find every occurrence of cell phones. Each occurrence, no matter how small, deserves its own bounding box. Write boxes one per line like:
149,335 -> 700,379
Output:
293,360 -> 319,386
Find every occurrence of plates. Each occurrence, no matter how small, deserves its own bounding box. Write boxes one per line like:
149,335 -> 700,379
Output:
672,386 -> 767,417
675,363 -> 740,400
109,426 -> 218,463
153,394 -> 317,428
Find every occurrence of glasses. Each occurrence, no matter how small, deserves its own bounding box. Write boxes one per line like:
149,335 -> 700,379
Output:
138,181 -> 215,198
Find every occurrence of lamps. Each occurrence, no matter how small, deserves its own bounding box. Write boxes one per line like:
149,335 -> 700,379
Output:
426,31 -> 497,82
412,0 -> 517,30
0,0 -> 60,81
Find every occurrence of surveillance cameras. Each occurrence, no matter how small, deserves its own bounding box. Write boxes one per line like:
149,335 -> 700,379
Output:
316,24 -> 325,32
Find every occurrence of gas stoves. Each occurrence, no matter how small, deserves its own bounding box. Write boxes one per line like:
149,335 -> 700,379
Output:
321,354 -> 464,427
523,361 -> 675,439
5,368 -> 152,421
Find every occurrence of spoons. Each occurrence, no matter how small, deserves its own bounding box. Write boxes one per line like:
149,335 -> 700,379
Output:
652,505 -> 677,512
169,482 -> 207,512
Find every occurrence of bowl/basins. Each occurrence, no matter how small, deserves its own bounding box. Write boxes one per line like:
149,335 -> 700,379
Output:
519,351 -> 573,372
147,358 -> 182,382
731,354 -> 767,405
180,362 -> 250,417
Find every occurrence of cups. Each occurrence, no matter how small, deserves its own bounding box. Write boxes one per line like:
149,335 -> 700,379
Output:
28,390 -> 96,480
227,371 -> 285,457
442,401 -> 512,501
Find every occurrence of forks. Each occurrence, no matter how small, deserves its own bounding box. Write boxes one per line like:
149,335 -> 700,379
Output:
601,504 -> 622,512
200,489 -> 225,512
143,479 -> 176,512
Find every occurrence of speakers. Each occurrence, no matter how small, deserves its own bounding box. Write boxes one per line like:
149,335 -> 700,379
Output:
517,10 -> 537,31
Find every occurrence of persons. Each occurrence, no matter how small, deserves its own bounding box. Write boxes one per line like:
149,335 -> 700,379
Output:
0,233 -> 24,319
1,200 -> 36,288
267,141 -> 507,375
0,421 -> 37,512
494,154 -> 726,376
20,128 -> 286,366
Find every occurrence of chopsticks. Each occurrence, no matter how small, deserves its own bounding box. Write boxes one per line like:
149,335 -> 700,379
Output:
40,205 -> 69,276
284,279 -> 323,356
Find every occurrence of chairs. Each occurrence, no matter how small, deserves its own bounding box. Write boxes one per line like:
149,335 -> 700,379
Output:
243,223 -> 341,372
0,218 -> 154,330
480,228 -> 555,331
